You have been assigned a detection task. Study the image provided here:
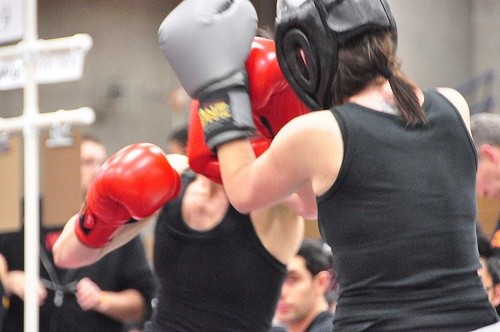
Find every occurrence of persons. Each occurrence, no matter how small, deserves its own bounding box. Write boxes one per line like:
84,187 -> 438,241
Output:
470,112 -> 500,200
0,133 -> 156,332
52,28 -> 313,332
275,237 -> 335,332
157,0 -> 500,332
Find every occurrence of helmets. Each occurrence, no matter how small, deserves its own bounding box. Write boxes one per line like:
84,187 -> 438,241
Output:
275,0 -> 397,109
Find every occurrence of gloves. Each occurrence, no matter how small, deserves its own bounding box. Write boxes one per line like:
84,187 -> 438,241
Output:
74,143 -> 182,249
157,0 -> 258,152
188,36 -> 312,188
